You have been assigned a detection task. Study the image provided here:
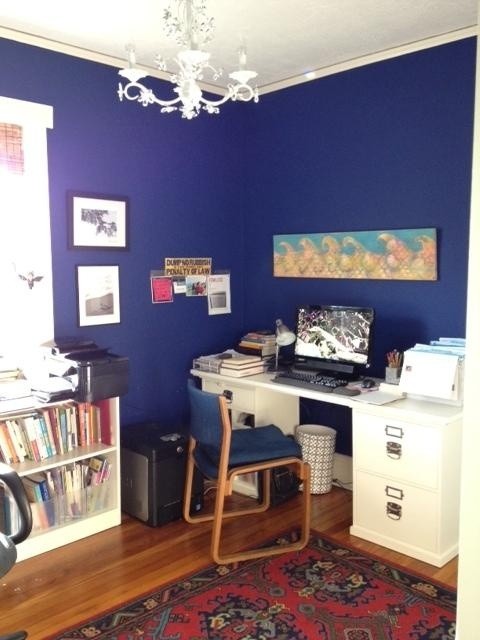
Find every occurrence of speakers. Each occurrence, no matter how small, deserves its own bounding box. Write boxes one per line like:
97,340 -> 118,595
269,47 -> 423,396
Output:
121,421 -> 203,528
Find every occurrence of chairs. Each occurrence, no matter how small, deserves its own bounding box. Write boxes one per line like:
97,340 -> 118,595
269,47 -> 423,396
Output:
182,377 -> 313,565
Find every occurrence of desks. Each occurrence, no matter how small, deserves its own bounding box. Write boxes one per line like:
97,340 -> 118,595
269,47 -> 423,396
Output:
188,365 -> 464,569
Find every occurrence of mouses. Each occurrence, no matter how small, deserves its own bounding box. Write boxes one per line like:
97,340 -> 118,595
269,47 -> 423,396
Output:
360,377 -> 375,388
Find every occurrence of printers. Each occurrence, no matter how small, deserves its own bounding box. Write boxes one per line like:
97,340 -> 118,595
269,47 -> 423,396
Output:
30,338 -> 129,403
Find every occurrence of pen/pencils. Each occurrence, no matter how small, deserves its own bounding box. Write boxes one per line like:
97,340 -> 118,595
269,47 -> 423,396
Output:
385,349 -> 402,368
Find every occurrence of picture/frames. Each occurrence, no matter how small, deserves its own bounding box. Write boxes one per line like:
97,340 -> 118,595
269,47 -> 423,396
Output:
65,189 -> 131,253
75,263 -> 122,328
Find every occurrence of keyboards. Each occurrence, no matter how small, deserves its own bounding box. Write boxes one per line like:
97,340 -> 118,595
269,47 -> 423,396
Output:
277,371 -> 348,388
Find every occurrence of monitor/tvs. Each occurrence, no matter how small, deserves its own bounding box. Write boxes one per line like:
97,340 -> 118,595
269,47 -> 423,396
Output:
294,305 -> 376,382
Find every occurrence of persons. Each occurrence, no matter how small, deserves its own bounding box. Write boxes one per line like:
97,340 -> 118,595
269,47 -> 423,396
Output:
196,282 -> 205,295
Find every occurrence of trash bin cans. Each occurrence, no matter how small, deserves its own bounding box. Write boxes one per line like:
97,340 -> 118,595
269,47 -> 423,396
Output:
296,424 -> 337,495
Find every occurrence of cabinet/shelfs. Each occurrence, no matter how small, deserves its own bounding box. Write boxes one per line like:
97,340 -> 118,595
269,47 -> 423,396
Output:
0,396 -> 122,565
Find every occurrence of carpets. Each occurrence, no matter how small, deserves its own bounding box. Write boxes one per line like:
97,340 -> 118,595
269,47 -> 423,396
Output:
42,526 -> 457,640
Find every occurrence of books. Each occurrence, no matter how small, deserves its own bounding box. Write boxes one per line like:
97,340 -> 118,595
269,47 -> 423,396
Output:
193,330 -> 276,377
398,337 -> 465,407
0,366 -> 117,536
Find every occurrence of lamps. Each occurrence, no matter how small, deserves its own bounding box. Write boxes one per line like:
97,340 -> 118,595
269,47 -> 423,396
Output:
266,318 -> 298,376
116,2 -> 260,121
